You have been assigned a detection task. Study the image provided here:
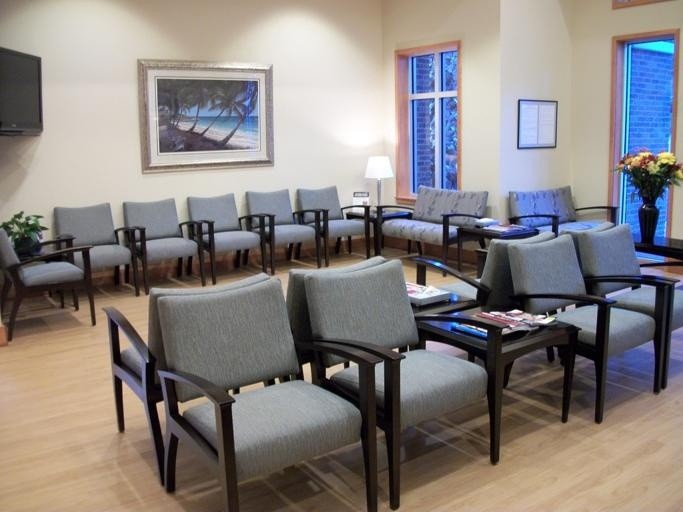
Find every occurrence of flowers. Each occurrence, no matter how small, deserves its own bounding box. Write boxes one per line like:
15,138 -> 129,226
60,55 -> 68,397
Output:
607,145 -> 682,202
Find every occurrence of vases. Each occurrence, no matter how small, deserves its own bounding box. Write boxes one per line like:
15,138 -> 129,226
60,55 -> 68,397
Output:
636,201 -> 659,245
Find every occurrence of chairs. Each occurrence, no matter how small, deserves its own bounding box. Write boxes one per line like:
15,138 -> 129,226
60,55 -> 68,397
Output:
51,200 -> 140,312
122,198 -> 208,295
294,185 -> 373,267
243,187 -> 323,275
304,259 -> 506,510
101,271 -> 269,490
184,193 -> 268,285
412,231 -> 556,310
0,225 -> 96,342
157,275 -> 383,511
280,252 -> 386,383
577,222 -> 681,389
505,234 -> 672,424
559,221 -> 615,266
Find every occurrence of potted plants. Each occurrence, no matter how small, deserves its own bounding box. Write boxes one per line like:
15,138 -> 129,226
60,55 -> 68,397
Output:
0,210 -> 50,256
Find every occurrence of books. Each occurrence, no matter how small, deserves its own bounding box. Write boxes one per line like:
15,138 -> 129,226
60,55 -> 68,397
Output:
449,308 -> 557,343
482,224 -> 528,233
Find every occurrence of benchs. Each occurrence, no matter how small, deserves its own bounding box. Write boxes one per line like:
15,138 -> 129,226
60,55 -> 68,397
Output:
374,184 -> 491,277
506,183 -> 618,237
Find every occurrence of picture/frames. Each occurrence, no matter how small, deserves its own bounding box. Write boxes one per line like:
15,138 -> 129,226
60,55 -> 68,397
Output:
515,97 -> 558,151
135,58 -> 275,176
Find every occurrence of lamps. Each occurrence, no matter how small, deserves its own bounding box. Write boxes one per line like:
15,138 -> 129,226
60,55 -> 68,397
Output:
362,154 -> 394,213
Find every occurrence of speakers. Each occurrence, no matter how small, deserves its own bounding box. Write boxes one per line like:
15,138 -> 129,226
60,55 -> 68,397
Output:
0,46 -> 43,136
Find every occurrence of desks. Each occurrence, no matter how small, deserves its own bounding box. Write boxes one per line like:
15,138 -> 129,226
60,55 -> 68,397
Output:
418,304 -> 583,466
343,211 -> 415,258
632,232 -> 683,268
398,279 -> 478,356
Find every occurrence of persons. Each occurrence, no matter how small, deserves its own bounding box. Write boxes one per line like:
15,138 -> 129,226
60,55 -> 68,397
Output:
404,282 -> 452,307
